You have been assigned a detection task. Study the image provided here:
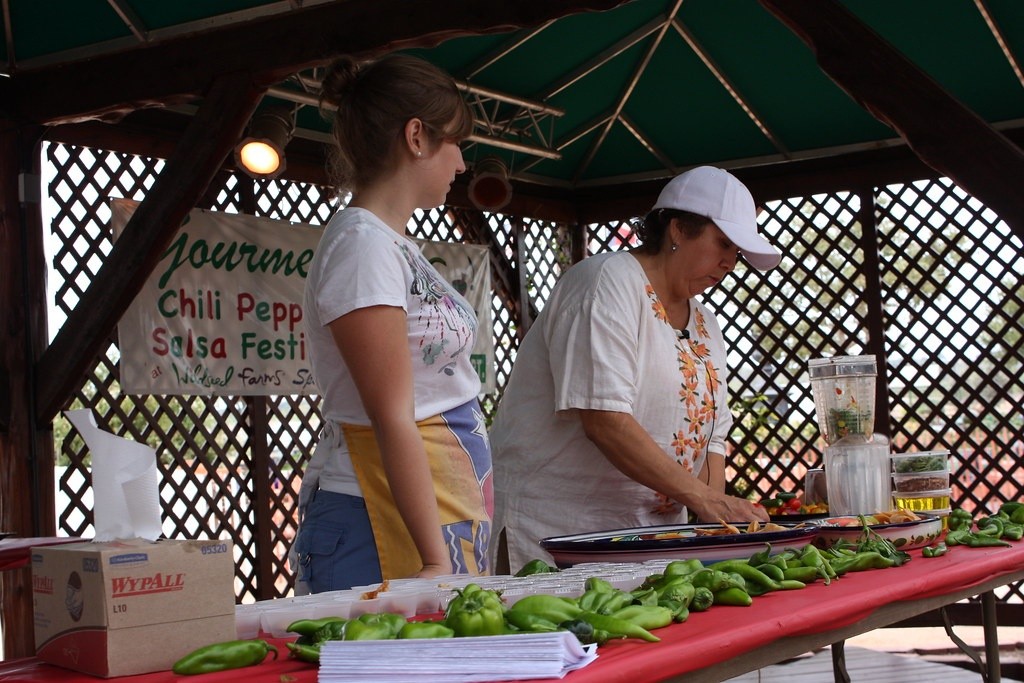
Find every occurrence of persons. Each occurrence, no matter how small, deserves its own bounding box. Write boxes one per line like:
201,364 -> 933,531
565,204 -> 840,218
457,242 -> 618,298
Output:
489,166 -> 782,576
290,51 -> 496,594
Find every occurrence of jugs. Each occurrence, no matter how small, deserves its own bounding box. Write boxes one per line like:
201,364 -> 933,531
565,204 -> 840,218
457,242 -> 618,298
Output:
825,433 -> 891,516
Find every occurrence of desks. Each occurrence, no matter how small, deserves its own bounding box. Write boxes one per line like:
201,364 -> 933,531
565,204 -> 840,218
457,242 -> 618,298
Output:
0,534 -> 1024,683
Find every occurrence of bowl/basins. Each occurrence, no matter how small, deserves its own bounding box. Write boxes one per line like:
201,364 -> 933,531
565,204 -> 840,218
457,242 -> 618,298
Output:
769,513 -> 828,524
235,559 -> 685,641
538,523 -> 820,569
926,510 -> 952,531
889,450 -> 952,471
802,512 -> 941,551
892,470 -> 951,491
891,489 -> 953,510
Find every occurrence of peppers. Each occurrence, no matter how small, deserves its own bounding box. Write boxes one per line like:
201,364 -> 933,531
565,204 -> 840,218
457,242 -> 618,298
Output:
172,492 -> 1024,678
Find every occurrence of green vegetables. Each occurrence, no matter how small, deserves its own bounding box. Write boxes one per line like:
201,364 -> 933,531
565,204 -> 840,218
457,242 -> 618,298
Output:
894,454 -> 945,474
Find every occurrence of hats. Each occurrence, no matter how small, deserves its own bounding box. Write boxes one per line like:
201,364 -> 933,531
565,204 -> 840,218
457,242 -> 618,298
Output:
652,165 -> 782,271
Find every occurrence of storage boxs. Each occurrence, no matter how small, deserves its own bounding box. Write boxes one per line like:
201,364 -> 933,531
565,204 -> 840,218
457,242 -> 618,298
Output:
888,450 -> 952,531
32,538 -> 235,677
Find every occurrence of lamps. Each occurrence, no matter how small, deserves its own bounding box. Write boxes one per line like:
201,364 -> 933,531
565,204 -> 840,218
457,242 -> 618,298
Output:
468,145 -> 515,212
235,102 -> 297,177
